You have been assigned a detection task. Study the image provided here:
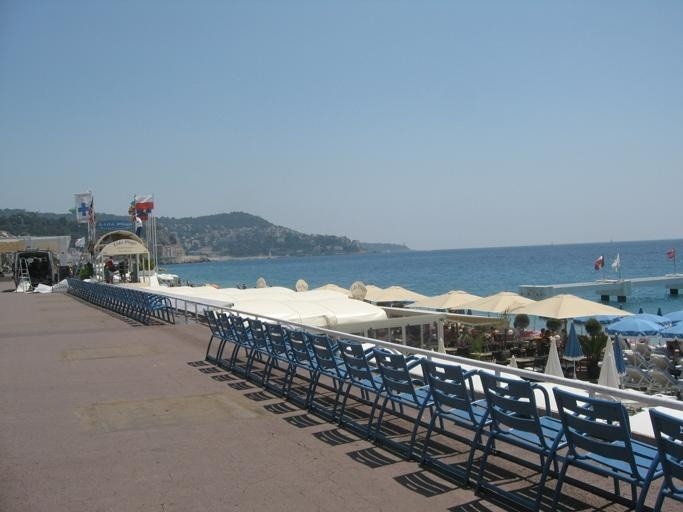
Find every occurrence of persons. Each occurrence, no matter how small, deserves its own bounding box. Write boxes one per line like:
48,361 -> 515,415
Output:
445,323 -> 567,354
133,215 -> 142,238
104,255 -> 116,284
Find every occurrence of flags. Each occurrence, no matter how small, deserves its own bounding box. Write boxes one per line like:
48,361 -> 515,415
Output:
125,192 -> 154,222
611,251 -> 622,274
665,247 -> 675,261
87,195 -> 96,226
73,190 -> 92,224
592,253 -> 604,272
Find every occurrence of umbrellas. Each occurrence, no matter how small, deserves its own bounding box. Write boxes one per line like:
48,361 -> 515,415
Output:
403,290 -> 484,337
561,322 -> 589,379
509,293 -> 635,341
456,291 -> 537,317
315,283 -> 347,298
546,336 -> 565,379
610,334 -> 627,380
597,335 -> 622,389
347,282 -> 383,299
363,284 -> 429,308
572,304 -> 683,368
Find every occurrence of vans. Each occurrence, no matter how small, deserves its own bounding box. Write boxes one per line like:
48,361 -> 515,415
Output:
11,247 -> 82,288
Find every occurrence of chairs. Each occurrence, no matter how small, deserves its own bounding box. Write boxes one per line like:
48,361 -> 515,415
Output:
204,310 -> 341,411
371,342 -> 488,462
416,353 -> 561,489
305,331 -> 373,418
637,403 -> 683,511
66,276 -> 175,326
546,384 -> 666,510
471,363 -> 582,510
359,308 -> 682,409
336,340 -> 411,439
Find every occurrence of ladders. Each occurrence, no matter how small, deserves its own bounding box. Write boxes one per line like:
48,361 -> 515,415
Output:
18,257 -> 33,292
74,255 -> 88,277
